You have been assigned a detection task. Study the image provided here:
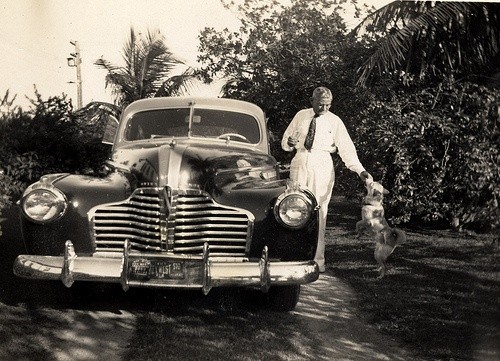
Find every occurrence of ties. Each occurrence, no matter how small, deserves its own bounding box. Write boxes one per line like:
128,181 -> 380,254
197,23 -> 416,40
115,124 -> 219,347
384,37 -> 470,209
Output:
305,114 -> 319,151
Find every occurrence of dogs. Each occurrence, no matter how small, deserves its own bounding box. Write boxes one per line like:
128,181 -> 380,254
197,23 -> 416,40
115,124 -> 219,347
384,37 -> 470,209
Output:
355,177 -> 405,281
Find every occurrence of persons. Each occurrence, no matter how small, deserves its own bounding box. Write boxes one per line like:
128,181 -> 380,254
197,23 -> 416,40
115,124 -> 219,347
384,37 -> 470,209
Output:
282,85 -> 373,274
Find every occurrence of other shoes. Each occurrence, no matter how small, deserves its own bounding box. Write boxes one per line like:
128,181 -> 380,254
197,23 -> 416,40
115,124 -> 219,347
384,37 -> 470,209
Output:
319,264 -> 325,272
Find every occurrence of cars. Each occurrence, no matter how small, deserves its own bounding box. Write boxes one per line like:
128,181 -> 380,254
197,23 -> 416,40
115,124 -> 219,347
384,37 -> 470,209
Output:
12,97 -> 322,315
